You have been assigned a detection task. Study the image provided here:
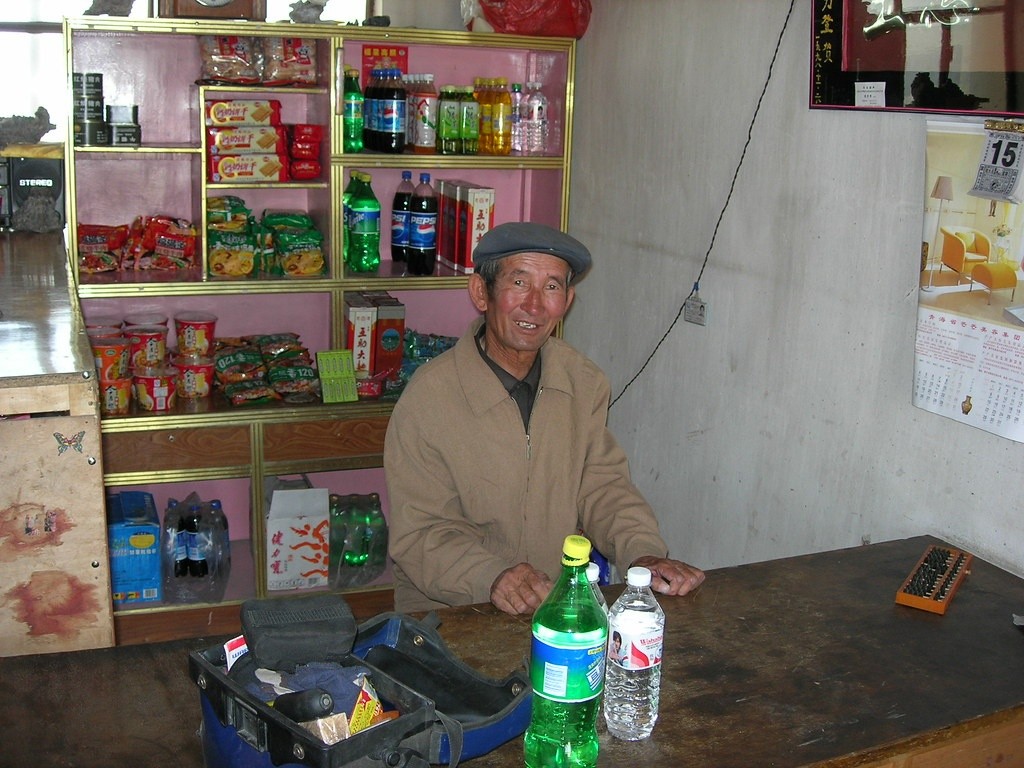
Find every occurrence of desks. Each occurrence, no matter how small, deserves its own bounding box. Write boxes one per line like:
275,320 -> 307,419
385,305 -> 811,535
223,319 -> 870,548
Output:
0,531 -> 1024,768
0,224 -> 117,659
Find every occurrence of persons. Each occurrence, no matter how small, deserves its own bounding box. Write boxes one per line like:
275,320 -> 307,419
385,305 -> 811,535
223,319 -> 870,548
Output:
384,221 -> 707,613
610,633 -> 630,668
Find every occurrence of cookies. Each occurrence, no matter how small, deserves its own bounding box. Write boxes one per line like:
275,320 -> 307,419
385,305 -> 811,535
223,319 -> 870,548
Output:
259,159 -> 283,178
251,103 -> 273,122
197,36 -> 317,84
257,131 -> 280,149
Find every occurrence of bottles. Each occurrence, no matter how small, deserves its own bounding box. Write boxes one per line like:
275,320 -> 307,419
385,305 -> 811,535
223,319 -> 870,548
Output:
605,566 -> 664,741
167,500 -> 229,577
343,168 -> 437,274
342,64 -> 548,155
522,535 -> 608,768
586,562 -> 609,620
328,494 -> 386,569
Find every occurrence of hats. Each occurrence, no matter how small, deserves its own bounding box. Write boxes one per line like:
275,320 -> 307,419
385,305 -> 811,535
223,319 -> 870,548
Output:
472,222 -> 592,282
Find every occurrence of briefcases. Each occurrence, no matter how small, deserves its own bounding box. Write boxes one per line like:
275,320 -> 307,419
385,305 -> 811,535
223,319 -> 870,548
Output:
185,591 -> 533,768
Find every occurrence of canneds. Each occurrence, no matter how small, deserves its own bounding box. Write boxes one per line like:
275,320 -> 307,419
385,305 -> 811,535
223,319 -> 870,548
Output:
70,72 -> 111,146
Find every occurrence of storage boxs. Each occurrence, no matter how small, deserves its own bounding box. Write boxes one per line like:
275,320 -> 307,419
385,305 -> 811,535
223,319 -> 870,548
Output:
105,492 -> 164,607
189,609 -> 533,768
434,178 -> 495,277
260,472 -> 332,592
343,290 -> 404,381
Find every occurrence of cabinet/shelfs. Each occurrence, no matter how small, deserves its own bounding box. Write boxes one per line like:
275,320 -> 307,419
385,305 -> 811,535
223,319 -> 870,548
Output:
61,12 -> 578,648
